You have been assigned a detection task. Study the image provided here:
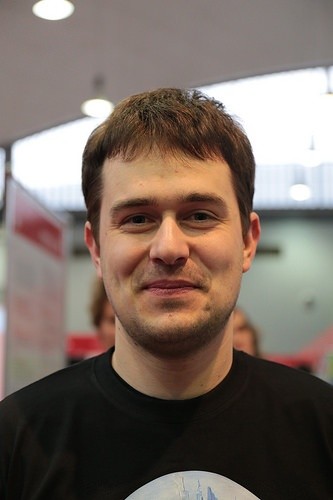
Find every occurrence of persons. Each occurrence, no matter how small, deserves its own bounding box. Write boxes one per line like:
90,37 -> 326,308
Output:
84,274 -> 116,360
230,308 -> 259,357
1,87 -> 333,499
